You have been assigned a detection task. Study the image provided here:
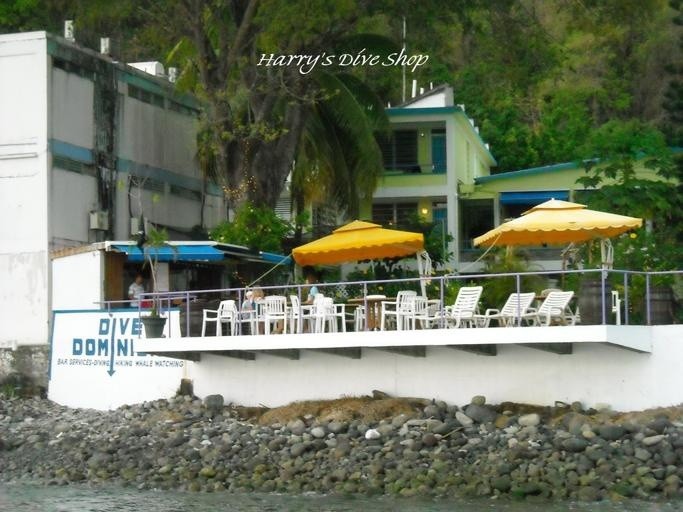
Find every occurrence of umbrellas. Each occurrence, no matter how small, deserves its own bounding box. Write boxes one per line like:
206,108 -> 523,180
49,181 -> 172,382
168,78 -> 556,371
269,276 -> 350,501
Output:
290,219 -> 432,330
473,197 -> 642,293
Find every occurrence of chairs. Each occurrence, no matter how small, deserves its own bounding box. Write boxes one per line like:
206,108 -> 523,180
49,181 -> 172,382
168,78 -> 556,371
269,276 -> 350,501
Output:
200,284 -> 574,336
610,289 -> 621,326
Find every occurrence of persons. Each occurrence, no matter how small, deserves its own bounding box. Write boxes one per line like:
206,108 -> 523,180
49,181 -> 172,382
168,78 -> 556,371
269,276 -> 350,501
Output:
303,275 -> 319,305
241,284 -> 265,335
127,275 -> 145,307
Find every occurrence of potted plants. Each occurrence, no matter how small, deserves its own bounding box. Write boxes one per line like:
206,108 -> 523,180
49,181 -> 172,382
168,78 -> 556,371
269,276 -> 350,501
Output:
629,263 -> 678,324
579,265 -> 612,325
117,173 -> 180,337
